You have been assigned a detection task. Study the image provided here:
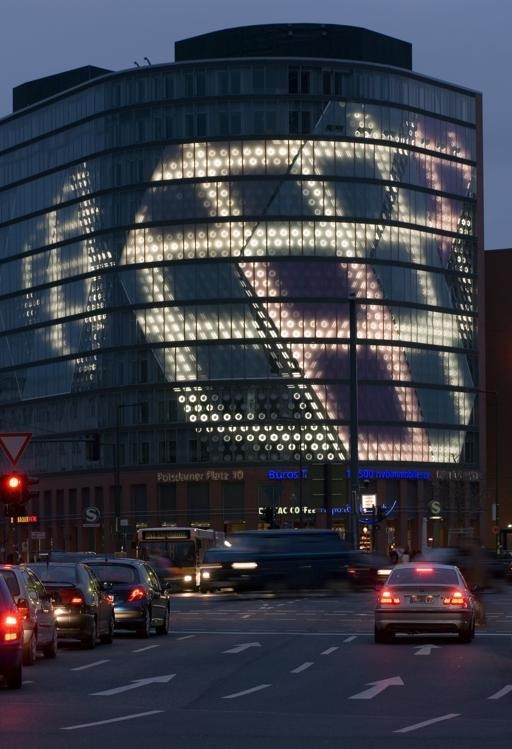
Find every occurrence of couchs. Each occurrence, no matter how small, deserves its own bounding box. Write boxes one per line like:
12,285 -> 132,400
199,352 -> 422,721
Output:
0,472 -> 30,505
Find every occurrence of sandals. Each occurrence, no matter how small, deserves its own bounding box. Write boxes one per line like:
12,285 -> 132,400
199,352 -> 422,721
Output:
136,527 -> 226,594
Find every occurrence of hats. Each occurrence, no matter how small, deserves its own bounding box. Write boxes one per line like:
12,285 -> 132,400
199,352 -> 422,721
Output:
200,527 -> 371,593
374,545 -> 504,642
0,551 -> 170,689
